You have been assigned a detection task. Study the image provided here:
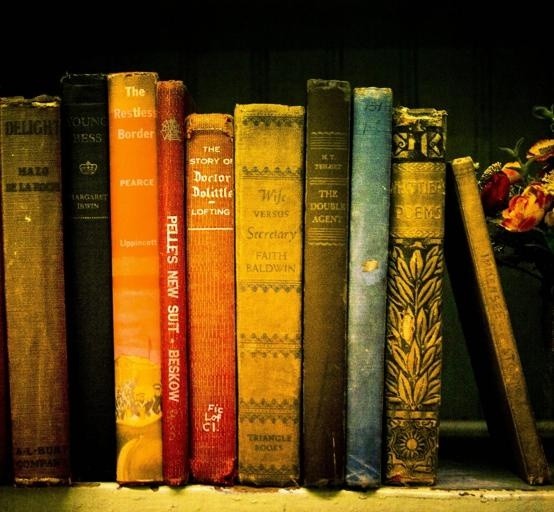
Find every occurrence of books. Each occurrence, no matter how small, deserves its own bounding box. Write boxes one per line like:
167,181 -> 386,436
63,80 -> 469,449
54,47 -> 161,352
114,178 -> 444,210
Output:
301,78 -> 351,487
154,79 -> 187,485
343,87 -> 393,490
450,156 -> 553,484
0,97 -> 70,485
106,72 -> 162,486
234,104 -> 307,486
61,74 -> 116,482
187,114 -> 235,483
385,108 -> 448,487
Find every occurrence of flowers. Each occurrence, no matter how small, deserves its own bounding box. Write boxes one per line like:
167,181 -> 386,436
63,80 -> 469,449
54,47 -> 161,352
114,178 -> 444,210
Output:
468,102 -> 554,276
